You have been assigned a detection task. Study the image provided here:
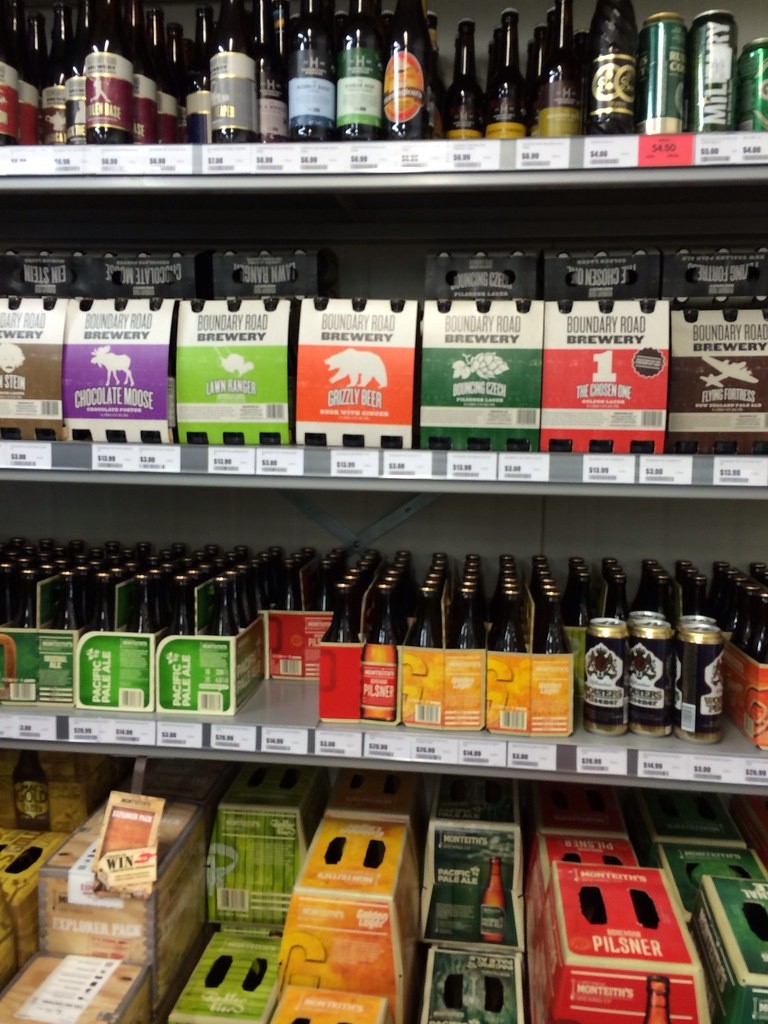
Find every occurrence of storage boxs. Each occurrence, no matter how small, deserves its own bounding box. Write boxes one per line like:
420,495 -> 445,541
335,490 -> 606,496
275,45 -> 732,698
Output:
0,292 -> 768,1024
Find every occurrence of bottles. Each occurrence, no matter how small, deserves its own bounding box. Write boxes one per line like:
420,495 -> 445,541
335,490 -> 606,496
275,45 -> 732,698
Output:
480,857 -> 505,942
643,976 -> 671,1024
0,0 -> 187,143
12,750 -> 51,831
277,545 -> 590,739
0,536 -> 277,759
189,0 -> 441,144
443,0 -> 641,140
594,555 -> 768,661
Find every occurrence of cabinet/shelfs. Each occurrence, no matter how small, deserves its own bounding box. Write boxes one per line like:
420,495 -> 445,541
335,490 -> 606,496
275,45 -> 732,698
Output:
0,132 -> 768,799
